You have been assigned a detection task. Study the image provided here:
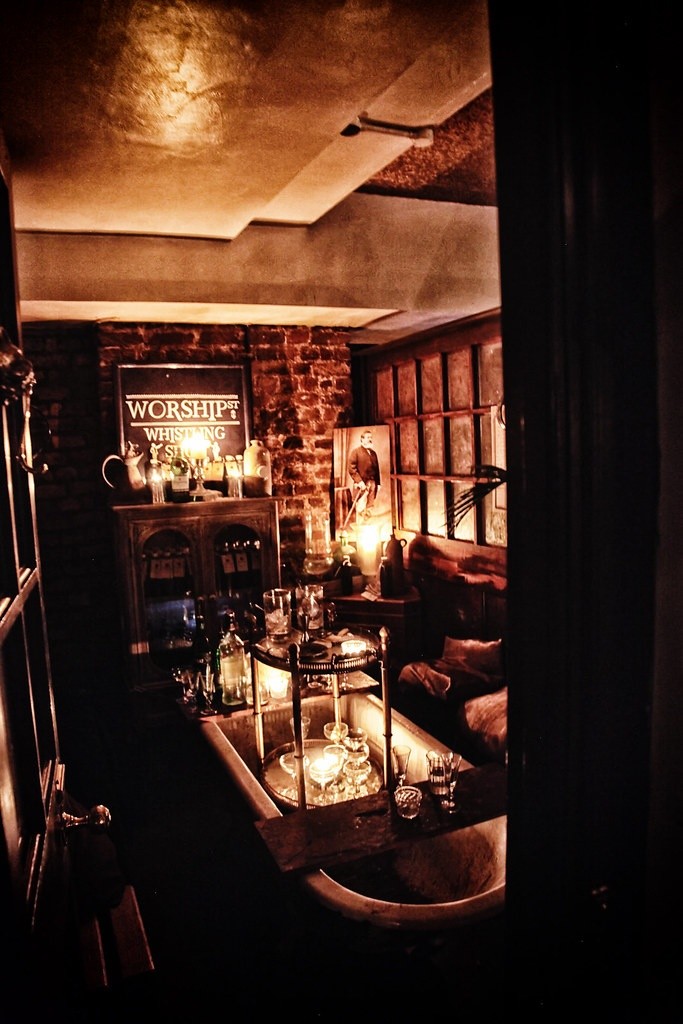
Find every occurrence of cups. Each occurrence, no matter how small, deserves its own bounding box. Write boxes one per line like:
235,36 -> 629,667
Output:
427,749 -> 448,795
263,588 -> 293,643
395,786 -> 422,819
295,585 -> 325,631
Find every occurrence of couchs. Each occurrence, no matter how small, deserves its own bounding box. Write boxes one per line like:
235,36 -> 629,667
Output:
399,574 -> 511,753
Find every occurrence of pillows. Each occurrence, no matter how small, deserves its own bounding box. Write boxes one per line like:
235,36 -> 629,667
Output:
434,635 -> 505,689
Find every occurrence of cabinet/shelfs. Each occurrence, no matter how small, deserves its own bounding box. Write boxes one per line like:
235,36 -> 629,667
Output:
108,494 -> 290,658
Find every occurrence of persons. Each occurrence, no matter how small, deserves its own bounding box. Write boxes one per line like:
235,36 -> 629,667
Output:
348,431 -> 381,524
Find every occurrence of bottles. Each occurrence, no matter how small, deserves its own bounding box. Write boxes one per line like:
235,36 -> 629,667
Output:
171,447 -> 193,504
141,544 -> 191,596
213,540 -> 260,589
246,614 -> 270,705
218,618 -> 245,706
341,555 -> 354,596
379,556 -> 394,598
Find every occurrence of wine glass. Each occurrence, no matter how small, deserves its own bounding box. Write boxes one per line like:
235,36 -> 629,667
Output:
165,635 -> 219,719
392,745 -> 411,789
436,752 -> 462,815
279,716 -> 371,805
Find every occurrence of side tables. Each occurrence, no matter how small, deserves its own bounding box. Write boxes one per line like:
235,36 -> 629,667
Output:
328,592 -> 421,644
